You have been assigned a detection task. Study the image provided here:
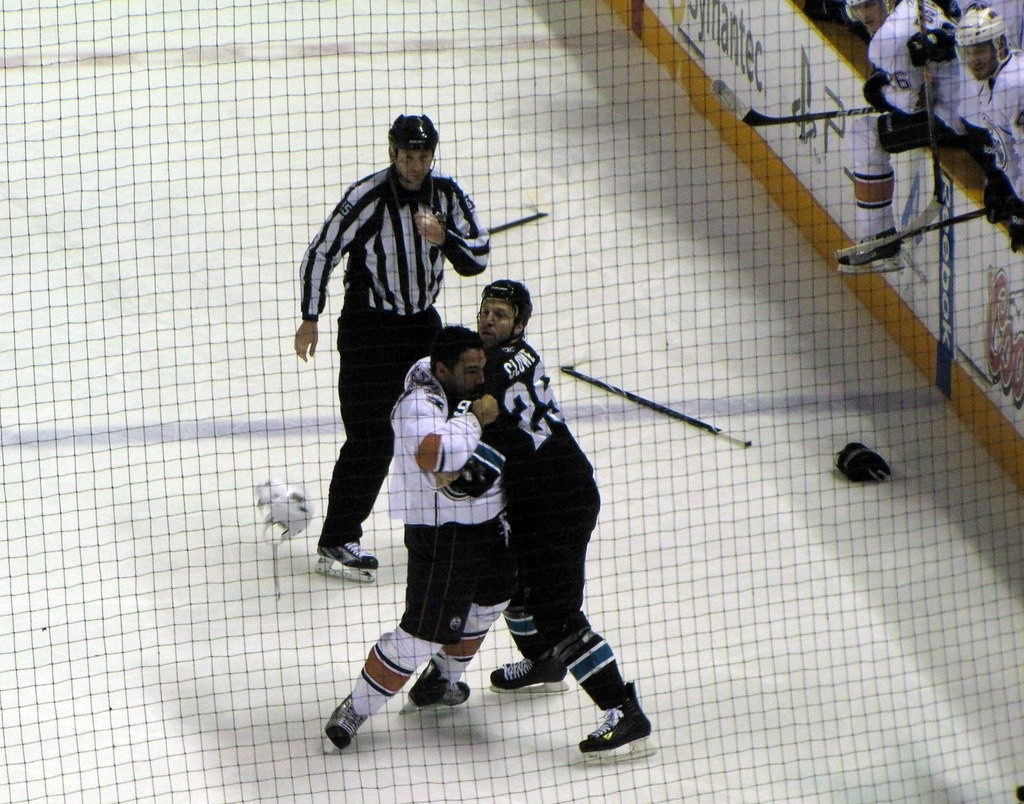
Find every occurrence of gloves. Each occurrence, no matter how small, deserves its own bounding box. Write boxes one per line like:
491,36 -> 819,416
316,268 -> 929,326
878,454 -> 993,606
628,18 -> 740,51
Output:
907,29 -> 957,67
863,68 -> 896,112
983,179 -> 1023,224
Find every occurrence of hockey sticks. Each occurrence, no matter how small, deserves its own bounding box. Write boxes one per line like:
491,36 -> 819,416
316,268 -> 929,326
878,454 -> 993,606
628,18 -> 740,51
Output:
560,363 -> 751,448
487,192 -> 550,236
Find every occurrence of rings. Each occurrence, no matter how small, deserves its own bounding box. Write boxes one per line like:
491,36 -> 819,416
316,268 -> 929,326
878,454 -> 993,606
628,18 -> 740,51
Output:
422,218 -> 431,225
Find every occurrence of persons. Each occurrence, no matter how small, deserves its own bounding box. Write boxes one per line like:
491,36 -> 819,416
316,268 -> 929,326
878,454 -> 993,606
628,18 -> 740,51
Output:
832,0 -> 1024,273
324,326 -> 513,752
294,116 -> 492,582
442,280 -> 656,765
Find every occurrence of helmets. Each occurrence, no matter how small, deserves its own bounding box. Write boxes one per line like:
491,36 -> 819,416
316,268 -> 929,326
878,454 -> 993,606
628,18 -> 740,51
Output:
845,0 -> 896,23
954,8 -> 1007,46
482,279 -> 532,329
388,114 -> 439,152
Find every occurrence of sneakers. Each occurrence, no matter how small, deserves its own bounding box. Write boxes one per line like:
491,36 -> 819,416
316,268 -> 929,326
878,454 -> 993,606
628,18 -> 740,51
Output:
489,653 -> 569,696
399,659 -> 471,716
578,680 -> 657,767
839,227 -> 905,276
315,540 -> 378,582
320,693 -> 367,754
